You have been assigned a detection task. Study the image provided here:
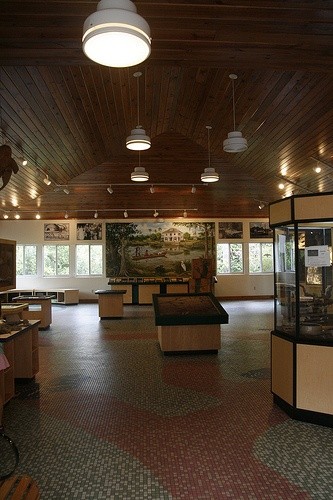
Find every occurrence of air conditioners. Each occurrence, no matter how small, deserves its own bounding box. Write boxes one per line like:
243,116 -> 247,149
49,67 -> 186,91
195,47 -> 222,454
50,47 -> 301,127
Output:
258,201 -> 266,209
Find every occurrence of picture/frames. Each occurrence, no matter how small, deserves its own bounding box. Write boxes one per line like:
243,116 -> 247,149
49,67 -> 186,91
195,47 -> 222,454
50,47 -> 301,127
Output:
76,223 -> 102,241
43,223 -> 70,241
249,220 -> 273,239
104,221 -> 219,283
217,221 -> 244,240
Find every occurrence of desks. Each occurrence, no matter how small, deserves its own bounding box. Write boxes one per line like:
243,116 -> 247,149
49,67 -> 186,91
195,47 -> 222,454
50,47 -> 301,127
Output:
151,292 -> 230,357
95,290 -> 128,319
0,288 -> 81,418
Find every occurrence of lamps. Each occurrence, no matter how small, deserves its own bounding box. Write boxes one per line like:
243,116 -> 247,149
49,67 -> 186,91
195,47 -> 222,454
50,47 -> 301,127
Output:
35,210 -> 41,220
80,0 -> 155,72
64,186 -> 70,196
19,156 -> 27,168
122,210 -> 129,218
2,212 -> 9,219
63,211 -> 70,219
149,185 -> 156,194
221,75 -> 251,154
14,212 -> 20,219
130,148 -> 150,182
43,175 -> 51,186
200,125 -> 220,184
93,210 -> 99,219
182,210 -> 188,217
190,185 -> 197,193
153,210 -> 159,218
106,185 -> 114,194
125,71 -> 152,153
316,162 -> 321,174
